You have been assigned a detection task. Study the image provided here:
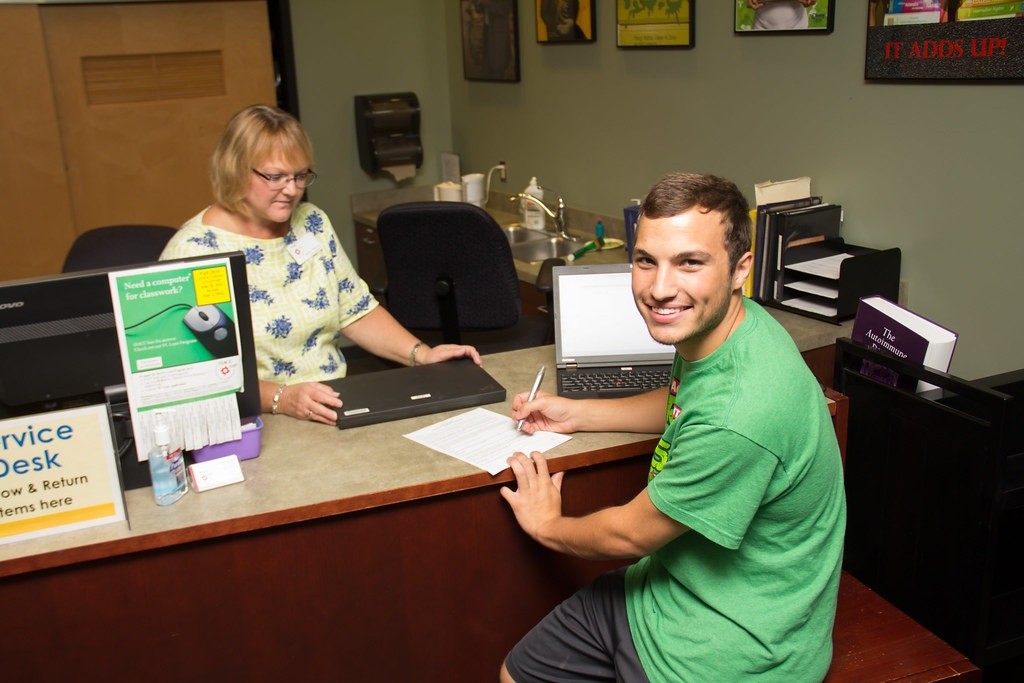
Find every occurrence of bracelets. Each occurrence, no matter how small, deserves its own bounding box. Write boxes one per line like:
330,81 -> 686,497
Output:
408,342 -> 428,366
271,384 -> 287,416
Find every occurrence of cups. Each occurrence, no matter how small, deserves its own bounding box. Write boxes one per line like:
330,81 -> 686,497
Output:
433,183 -> 461,202
461,172 -> 485,203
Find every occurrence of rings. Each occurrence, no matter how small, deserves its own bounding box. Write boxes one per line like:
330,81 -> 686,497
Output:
309,409 -> 312,416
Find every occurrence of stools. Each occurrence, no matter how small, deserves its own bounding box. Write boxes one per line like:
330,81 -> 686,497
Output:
824,570 -> 982,683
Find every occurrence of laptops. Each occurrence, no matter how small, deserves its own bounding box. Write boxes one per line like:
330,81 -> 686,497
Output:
552,263 -> 677,399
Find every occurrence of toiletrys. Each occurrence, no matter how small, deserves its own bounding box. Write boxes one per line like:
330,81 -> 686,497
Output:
147,415 -> 189,506
524,175 -> 546,230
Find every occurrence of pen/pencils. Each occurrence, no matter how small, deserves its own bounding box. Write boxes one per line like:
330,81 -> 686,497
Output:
517,366 -> 545,433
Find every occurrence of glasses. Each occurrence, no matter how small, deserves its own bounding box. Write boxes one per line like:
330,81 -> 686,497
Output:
252,167 -> 318,189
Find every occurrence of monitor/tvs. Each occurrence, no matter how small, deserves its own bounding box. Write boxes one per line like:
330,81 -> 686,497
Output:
0,251 -> 262,490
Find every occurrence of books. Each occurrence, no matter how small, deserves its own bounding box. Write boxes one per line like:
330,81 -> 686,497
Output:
851,294 -> 959,395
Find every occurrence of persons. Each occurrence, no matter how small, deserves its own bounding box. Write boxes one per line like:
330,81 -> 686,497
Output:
158,103 -> 483,427
500,169 -> 846,683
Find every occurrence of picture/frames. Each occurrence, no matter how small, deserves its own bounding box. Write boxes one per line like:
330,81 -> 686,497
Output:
615,0 -> 696,50
733,0 -> 835,34
459,0 -> 520,83
534,0 -> 596,44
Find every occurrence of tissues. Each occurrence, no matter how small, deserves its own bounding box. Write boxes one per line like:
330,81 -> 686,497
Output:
353,90 -> 424,182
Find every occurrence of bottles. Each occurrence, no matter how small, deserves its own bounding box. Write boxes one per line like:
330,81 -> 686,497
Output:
148,412 -> 188,506
524,176 -> 545,230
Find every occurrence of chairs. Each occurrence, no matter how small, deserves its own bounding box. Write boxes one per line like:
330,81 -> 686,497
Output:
377,200 -> 565,356
62,225 -> 179,274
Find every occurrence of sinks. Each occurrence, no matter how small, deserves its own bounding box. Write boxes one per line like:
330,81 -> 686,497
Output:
510,239 -> 585,264
500,224 -> 552,243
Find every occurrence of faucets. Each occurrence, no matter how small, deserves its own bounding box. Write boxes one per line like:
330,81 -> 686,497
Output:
508,194 -> 567,234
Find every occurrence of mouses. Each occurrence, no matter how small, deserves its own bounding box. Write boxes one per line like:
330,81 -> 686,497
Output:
182,304 -> 238,358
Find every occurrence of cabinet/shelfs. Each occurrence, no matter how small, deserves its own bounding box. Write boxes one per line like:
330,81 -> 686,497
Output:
353,221 -> 548,319
833,337 -> 1024,683
755,237 -> 901,326
